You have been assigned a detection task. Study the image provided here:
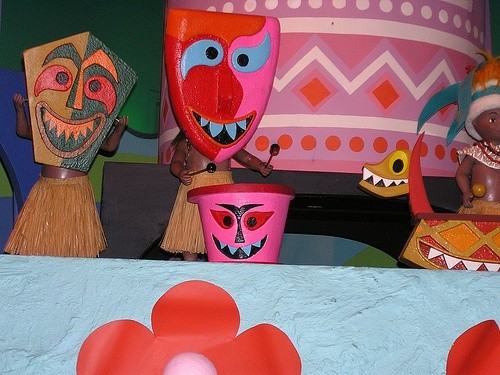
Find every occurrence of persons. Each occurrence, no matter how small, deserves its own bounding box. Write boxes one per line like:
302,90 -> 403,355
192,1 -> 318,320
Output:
455,105 -> 499,215
169,129 -> 274,262
11,94 -> 128,257
